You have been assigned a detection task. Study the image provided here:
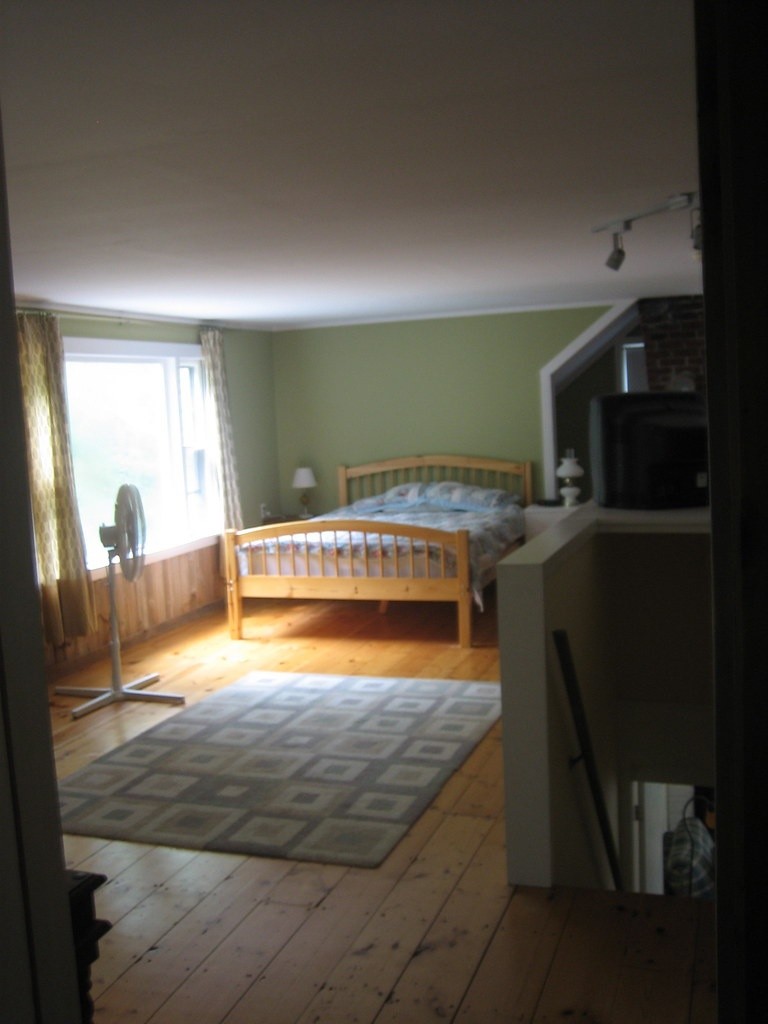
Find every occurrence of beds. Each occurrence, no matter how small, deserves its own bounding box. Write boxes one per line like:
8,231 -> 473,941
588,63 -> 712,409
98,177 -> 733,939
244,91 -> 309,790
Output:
225,454 -> 533,649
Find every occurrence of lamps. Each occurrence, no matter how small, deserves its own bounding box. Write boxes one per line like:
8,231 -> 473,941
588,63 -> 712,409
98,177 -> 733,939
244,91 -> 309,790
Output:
292,468 -> 319,520
590,192 -> 702,271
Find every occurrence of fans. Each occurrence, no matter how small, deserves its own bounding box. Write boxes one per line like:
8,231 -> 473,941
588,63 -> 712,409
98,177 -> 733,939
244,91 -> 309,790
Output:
55,483 -> 186,719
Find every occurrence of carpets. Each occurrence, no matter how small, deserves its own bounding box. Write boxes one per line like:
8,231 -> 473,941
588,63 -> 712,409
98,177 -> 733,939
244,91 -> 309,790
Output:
58,670 -> 501,869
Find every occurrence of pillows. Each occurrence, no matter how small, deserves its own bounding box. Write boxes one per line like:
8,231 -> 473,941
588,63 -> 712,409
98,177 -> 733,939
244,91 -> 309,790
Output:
418,482 -> 520,507
342,482 -> 424,514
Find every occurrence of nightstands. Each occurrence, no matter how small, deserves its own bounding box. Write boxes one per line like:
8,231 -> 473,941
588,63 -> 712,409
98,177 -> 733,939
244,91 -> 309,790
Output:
524,503 -> 584,544
260,514 -> 318,526
